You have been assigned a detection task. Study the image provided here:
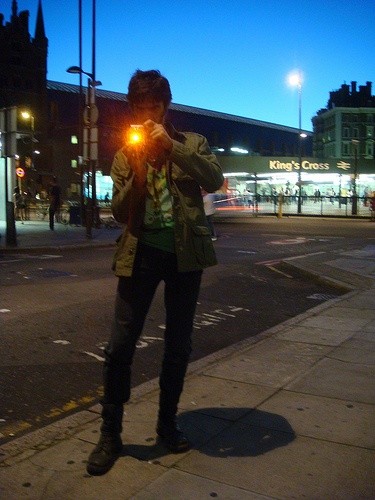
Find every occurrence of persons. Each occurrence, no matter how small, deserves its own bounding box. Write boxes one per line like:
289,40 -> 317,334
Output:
87,70 -> 225,476
12,176 -> 63,232
200,181 -> 375,240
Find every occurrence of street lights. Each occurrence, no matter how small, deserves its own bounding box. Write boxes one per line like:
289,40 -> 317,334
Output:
289,73 -> 304,215
66,65 -> 103,215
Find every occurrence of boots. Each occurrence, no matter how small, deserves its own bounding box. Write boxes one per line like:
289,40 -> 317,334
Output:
87,395 -> 126,473
155,378 -> 189,449
